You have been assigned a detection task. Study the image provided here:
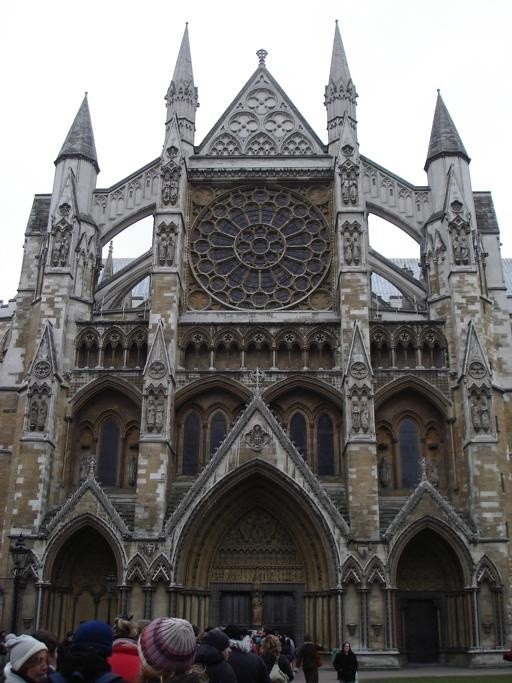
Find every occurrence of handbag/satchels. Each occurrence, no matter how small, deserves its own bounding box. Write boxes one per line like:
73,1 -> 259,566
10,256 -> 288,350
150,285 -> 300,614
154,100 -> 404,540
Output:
268,662 -> 289,683
315,655 -> 323,667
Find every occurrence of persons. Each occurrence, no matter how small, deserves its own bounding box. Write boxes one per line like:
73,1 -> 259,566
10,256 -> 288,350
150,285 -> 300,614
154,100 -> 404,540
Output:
331,640 -> 358,682
0,614 -> 296,682
294,631 -> 325,682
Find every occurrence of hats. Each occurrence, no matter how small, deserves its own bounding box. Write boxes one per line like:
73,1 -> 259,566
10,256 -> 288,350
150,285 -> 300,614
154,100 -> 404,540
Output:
5,632 -> 49,672
202,629 -> 230,651
136,617 -> 199,674
69,620 -> 114,657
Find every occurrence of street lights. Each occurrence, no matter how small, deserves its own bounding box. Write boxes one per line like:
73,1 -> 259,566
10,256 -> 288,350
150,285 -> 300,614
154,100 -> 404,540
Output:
104,563 -> 119,624
7,526 -> 36,634
